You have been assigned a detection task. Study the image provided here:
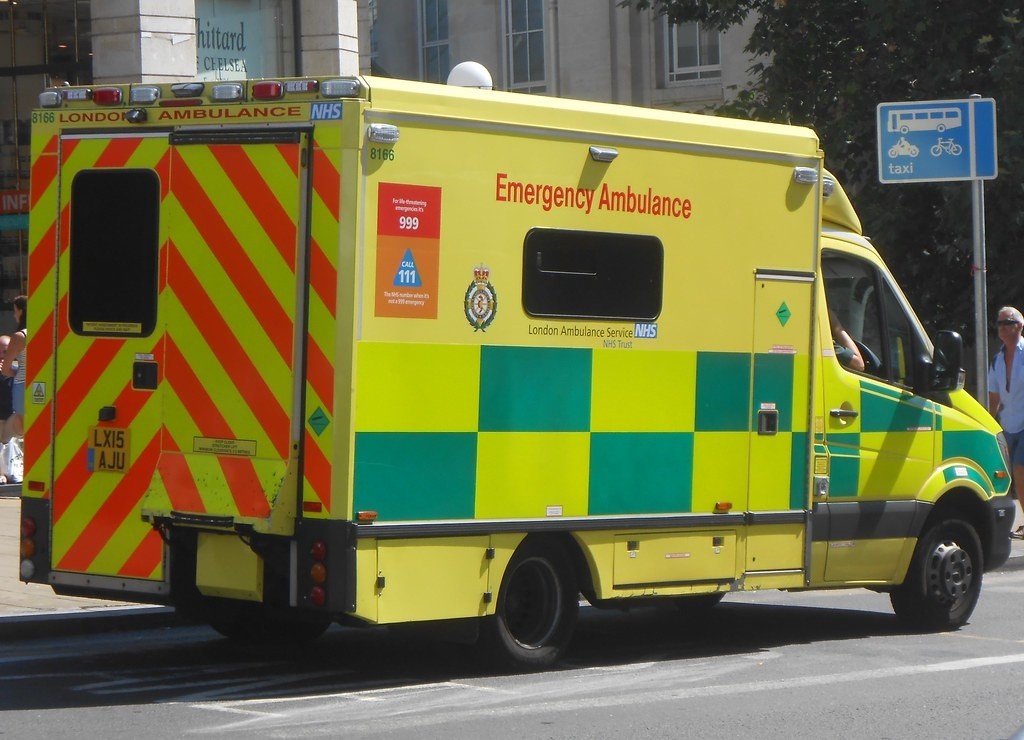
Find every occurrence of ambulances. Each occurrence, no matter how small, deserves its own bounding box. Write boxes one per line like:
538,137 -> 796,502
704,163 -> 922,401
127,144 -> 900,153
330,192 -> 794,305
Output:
19,77 -> 1016,671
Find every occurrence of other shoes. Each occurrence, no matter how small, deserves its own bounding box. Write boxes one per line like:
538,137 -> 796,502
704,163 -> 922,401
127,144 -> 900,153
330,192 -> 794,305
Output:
1011,525 -> 1024,539
0,475 -> 7,485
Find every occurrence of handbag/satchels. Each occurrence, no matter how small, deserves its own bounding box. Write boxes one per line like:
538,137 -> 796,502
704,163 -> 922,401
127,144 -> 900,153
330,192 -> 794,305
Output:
0,437 -> 24,483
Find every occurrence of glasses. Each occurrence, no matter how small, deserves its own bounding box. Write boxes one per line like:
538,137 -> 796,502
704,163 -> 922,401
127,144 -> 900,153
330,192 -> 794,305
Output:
997,319 -> 1020,326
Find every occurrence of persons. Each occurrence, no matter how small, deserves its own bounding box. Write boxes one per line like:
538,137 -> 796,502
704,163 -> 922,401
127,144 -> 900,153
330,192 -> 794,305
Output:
987,306 -> 1024,539
828,306 -> 865,371
0,296 -> 27,485
